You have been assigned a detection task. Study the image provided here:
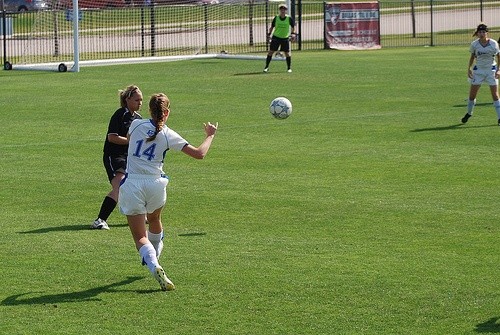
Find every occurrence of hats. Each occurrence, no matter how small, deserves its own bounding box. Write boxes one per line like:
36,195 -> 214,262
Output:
279,6 -> 287,10
477,24 -> 488,32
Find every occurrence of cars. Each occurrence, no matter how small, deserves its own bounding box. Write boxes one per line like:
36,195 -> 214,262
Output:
0,0 -> 48,14
52,0 -> 126,10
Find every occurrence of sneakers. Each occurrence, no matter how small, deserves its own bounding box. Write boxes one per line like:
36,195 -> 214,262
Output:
141,256 -> 147,266
498,119 -> 500,126
264,67 -> 269,72
91,218 -> 109,230
287,68 -> 292,73
154,267 -> 175,291
461,113 -> 472,123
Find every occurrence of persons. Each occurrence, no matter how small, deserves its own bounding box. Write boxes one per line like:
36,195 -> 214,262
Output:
263,4 -> 295,72
92,85 -> 148,229
118,93 -> 219,289
461,24 -> 500,125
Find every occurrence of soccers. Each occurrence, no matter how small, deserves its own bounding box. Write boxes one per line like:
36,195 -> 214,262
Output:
269,97 -> 294,120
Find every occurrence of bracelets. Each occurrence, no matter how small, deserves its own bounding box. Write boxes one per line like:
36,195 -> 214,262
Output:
291,32 -> 295,34
268,33 -> 270,35
